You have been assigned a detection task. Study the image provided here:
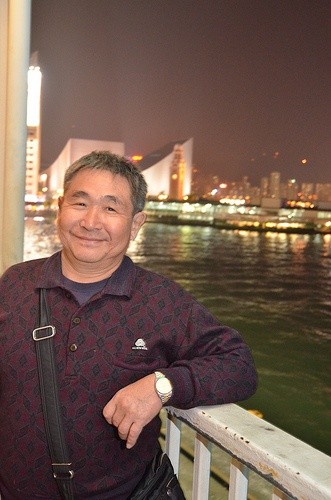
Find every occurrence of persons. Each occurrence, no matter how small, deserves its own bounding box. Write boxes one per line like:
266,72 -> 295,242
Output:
1,150 -> 261,500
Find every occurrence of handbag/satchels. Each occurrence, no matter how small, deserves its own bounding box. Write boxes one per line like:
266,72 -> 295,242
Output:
127,450 -> 187,500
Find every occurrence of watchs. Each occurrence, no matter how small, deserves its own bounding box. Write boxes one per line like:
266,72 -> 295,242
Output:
153,369 -> 173,406
